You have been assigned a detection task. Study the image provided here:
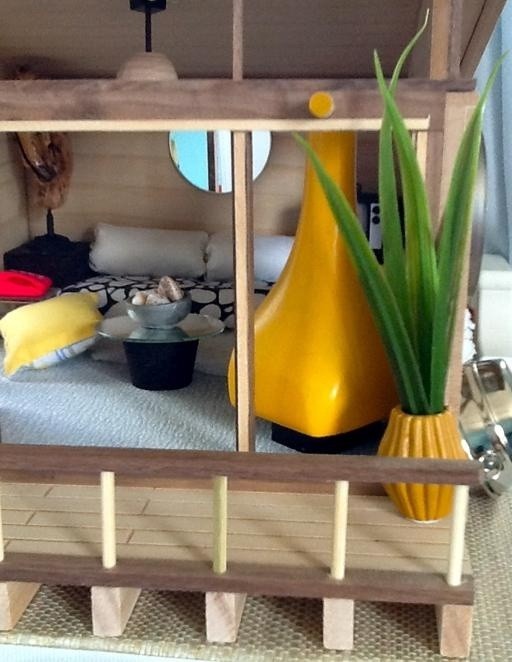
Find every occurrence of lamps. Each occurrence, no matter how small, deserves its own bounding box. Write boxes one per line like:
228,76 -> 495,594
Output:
117,1 -> 178,80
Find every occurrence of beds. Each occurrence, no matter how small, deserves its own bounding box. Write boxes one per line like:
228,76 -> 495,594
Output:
0,230 -> 380,456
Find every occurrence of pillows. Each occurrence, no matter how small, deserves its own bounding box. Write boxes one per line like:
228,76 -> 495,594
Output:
89,222 -> 210,280
0,270 -> 52,301
0,292 -> 102,376
206,231 -> 295,285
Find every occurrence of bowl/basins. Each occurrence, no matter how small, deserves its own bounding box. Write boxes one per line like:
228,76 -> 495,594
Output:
125,291 -> 193,329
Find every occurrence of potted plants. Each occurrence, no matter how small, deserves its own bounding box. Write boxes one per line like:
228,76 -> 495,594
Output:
276,7 -> 510,523
